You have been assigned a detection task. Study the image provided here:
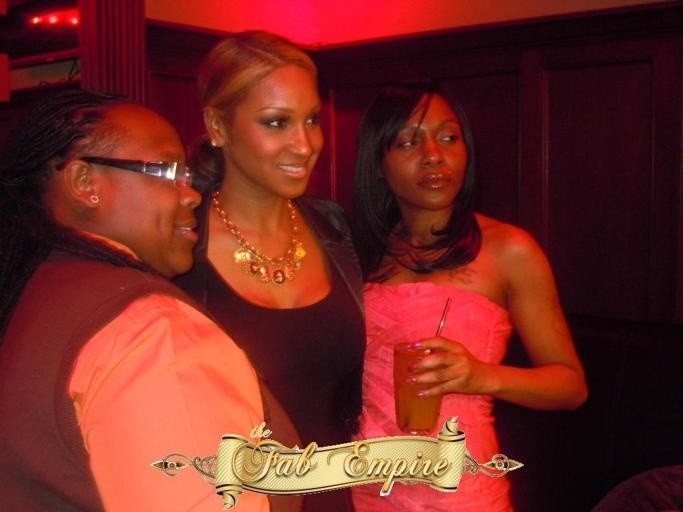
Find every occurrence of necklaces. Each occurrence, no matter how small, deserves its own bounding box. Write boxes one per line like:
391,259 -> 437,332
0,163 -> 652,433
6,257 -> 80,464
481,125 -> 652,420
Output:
213,194 -> 306,288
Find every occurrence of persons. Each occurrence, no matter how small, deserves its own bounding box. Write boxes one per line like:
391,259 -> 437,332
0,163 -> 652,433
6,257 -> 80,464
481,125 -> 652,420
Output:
351,79 -> 590,512
183,33 -> 368,512
0,91 -> 306,511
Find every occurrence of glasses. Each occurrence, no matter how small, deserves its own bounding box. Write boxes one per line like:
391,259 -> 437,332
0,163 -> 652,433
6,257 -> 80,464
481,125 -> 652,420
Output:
55,153 -> 196,190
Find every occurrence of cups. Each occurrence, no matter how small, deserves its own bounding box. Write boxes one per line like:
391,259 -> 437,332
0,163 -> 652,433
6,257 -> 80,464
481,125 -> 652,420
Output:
392,343 -> 443,433
